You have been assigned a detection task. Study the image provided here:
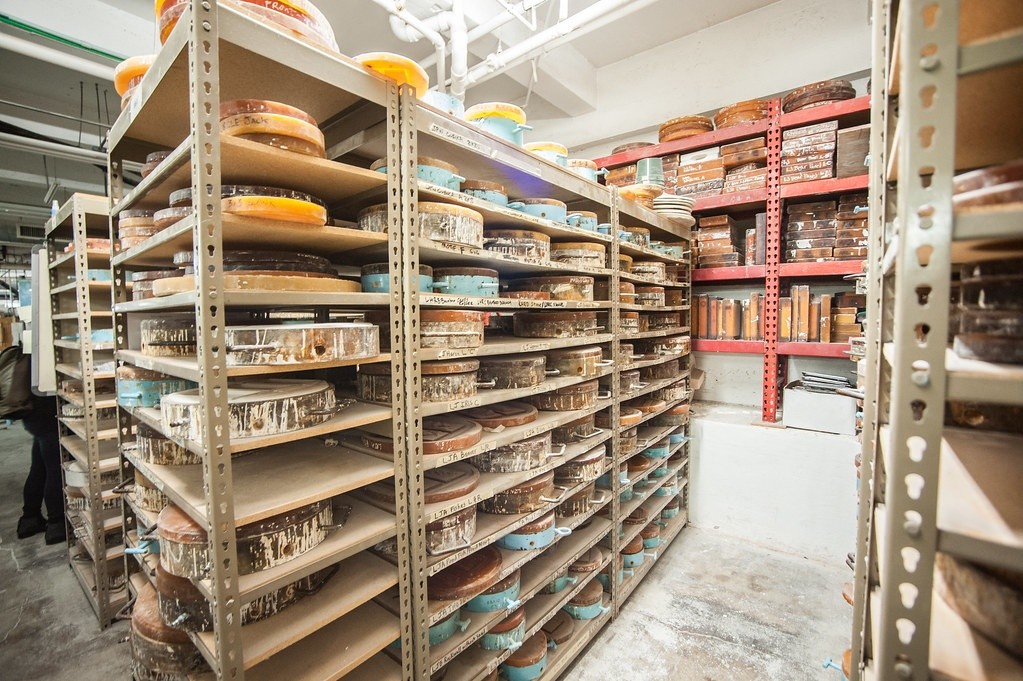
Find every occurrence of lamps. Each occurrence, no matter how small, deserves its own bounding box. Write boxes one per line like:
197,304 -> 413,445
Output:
43,154 -> 61,204
0,80 -> 145,173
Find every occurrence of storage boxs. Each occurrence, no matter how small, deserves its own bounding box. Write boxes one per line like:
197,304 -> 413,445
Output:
779,381 -> 858,436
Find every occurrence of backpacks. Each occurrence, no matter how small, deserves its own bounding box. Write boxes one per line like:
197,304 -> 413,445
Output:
0,345 -> 33,420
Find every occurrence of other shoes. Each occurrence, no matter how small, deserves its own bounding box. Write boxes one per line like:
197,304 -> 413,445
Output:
17,514 -> 47,538
45,522 -> 66,544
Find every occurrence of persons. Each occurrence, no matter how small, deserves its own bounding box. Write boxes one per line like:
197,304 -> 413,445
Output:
17,390 -> 76,545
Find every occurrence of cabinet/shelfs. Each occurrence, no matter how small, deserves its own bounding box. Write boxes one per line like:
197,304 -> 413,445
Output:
45,0 -> 1023,681
